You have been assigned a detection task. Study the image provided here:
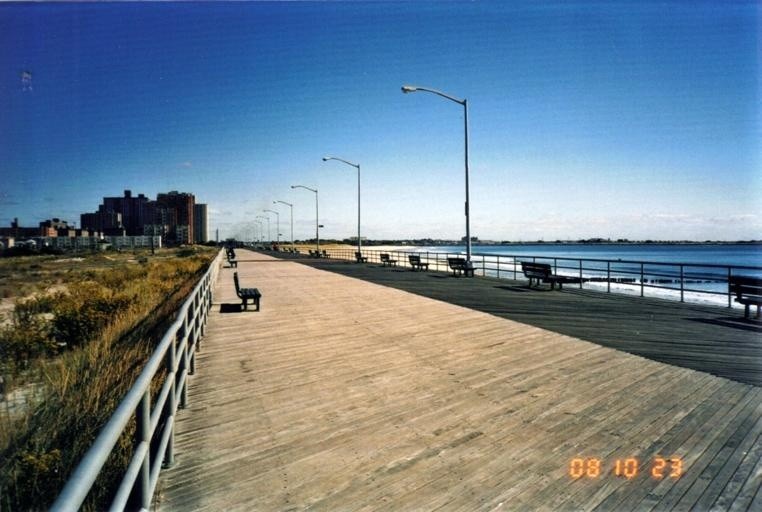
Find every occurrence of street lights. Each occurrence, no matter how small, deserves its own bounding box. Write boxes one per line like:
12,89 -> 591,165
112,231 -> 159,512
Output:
291,184 -> 319,251
322,156 -> 361,253
236,209 -> 280,253
274,201 -> 293,253
400,86 -> 474,278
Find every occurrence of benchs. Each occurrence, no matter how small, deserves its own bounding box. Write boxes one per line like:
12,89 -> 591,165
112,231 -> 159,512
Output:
234,272 -> 261,311
355,252 -> 368,262
727,274 -> 762,318
447,257 -> 477,277
283,247 -> 331,259
226,244 -> 238,268
380,254 -> 397,267
521,262 -> 564,290
408,256 -> 429,271
